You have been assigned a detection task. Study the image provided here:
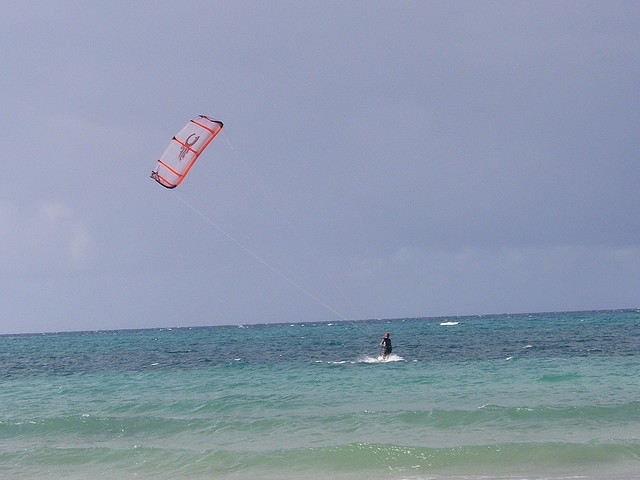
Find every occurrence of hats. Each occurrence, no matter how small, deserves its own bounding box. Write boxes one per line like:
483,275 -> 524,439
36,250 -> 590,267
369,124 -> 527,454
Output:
384,332 -> 389,337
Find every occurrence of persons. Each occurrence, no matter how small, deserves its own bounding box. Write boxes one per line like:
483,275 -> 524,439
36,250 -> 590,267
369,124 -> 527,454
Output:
379,332 -> 392,359
